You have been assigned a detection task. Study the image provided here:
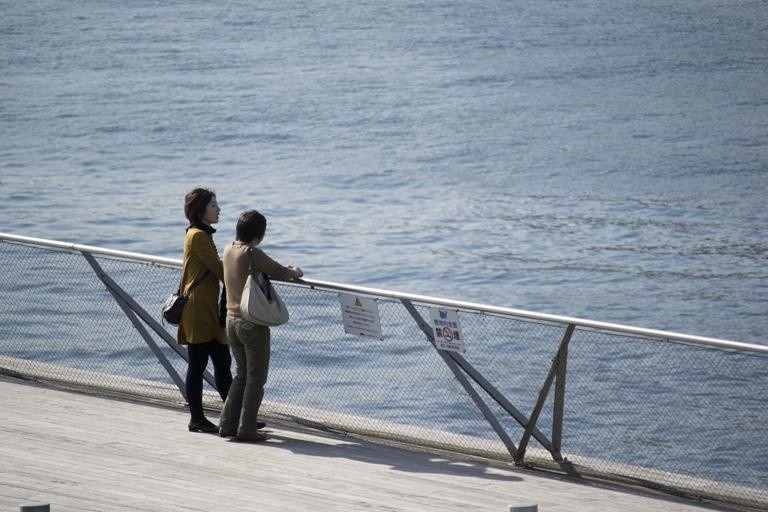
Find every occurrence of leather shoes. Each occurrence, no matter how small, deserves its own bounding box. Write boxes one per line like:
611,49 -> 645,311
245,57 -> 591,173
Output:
189,421 -> 266,442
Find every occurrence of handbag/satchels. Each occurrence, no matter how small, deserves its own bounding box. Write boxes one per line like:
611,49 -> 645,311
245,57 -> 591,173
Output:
240,249 -> 289,326
162,294 -> 184,324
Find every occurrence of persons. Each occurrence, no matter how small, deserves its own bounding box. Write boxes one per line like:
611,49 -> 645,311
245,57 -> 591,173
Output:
176,188 -> 304,443
